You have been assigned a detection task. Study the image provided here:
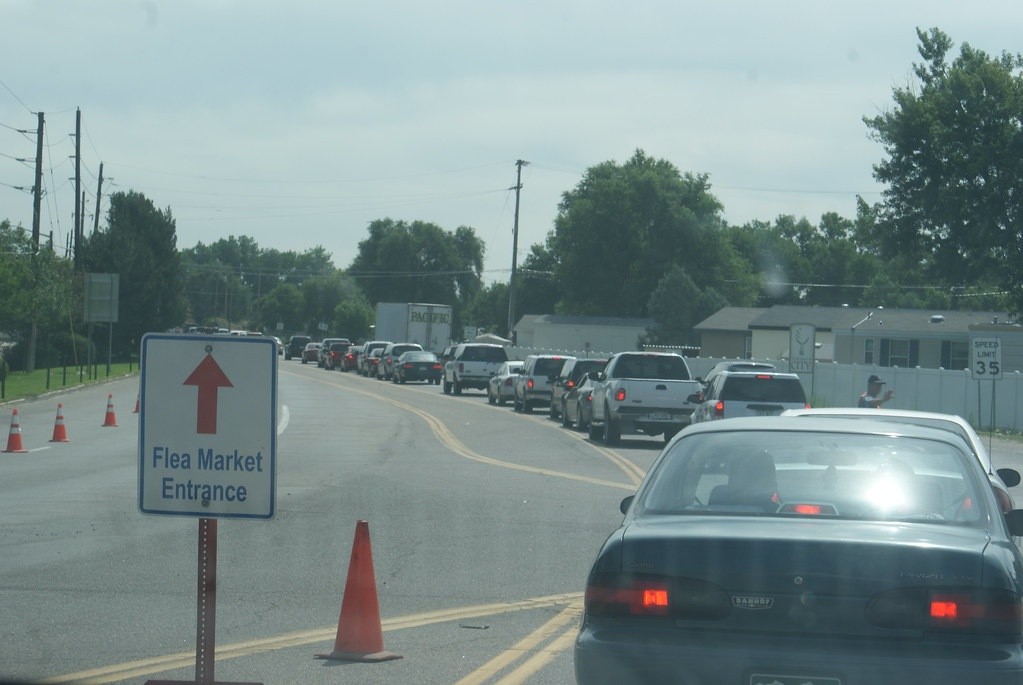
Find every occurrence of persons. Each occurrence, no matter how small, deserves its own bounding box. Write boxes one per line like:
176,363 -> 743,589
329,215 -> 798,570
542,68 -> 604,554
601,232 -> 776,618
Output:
858,375 -> 894,408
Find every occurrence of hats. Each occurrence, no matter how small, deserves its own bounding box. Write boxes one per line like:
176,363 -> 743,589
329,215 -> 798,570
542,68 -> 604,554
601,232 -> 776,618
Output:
868,374 -> 887,384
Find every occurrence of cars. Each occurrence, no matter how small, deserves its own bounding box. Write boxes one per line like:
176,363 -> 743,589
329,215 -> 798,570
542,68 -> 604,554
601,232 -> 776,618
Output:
572,416 -> 1023,685
779,407 -> 1017,515
694,357 -> 776,389
487,361 -> 525,405
181,324 -> 458,388
513,354 -> 576,414
560,371 -> 603,429
686,370 -> 811,424
587,351 -> 704,445
546,358 -> 610,419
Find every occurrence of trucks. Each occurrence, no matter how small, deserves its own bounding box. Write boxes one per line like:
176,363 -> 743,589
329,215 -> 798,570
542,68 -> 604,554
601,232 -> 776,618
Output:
368,302 -> 452,362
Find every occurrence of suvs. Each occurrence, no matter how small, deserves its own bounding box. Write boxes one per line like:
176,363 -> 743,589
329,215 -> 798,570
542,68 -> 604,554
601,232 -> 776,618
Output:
444,343 -> 508,395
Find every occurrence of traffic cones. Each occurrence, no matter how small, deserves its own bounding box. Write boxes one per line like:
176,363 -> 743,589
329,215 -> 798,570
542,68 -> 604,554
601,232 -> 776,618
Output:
48,403 -> 70,443
314,519 -> 404,664
132,385 -> 140,413
1,408 -> 29,454
101,393 -> 120,427
